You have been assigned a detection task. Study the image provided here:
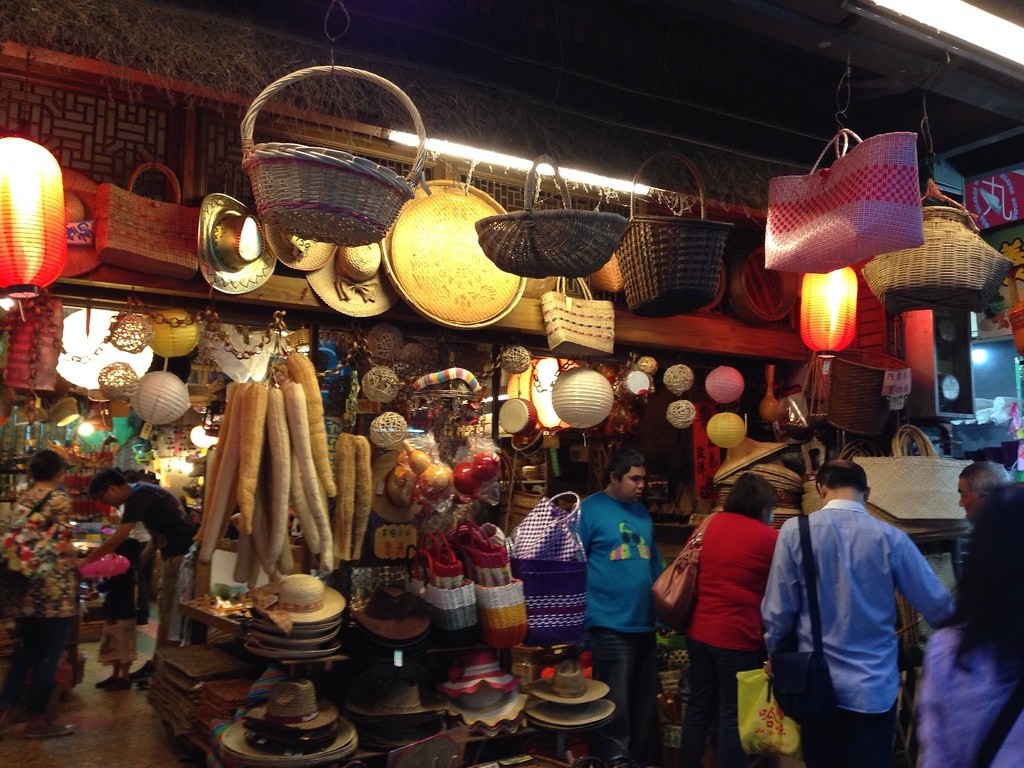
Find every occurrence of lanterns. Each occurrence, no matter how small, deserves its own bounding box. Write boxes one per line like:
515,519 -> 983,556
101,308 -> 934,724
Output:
132,370 -> 191,423
706,411 -> 746,447
552,367 -> 613,428
706,366 -> 745,402
4,297 -> 64,397
507,358 -> 581,436
0,137 -> 67,298
801,266 -> 858,358
145,308 -> 200,358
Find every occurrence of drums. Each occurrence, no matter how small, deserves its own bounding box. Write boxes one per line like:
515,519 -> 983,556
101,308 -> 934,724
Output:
499,398 -> 538,436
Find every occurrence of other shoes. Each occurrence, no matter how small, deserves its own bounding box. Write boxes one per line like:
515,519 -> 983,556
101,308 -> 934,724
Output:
0,720 -> 12,736
23,718 -> 73,739
93,674 -> 130,692
130,658 -> 155,681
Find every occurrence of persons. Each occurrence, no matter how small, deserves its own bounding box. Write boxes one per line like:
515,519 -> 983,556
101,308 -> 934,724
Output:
78,469 -> 193,690
918,482 -> 1024,768
761,458 -> 954,768
958,461 -> 1012,520
572,453 -> 672,768
0,449 -> 80,737
679,473 -> 778,768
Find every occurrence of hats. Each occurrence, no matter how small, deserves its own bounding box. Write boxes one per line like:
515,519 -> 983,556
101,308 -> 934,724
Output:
710,427 -> 805,531
197,192 -> 276,293
60,167 -> 106,277
306,228 -> 398,317
261,213 -> 338,271
217,568 -> 616,767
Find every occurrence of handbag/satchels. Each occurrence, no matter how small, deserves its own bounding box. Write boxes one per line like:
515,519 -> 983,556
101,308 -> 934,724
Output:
0,489 -> 74,579
765,130 -> 924,274
94,165 -> 198,280
541,271 -> 615,357
801,419 -> 979,657
651,510 -> 718,629
771,648 -> 835,721
408,488 -> 589,644
735,669 -> 803,760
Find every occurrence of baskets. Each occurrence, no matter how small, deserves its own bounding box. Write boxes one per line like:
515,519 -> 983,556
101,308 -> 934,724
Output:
860,189 -> 1013,312
239,66 -> 425,248
47,398 -> 80,426
613,149 -> 733,318
474,154 -> 629,277
